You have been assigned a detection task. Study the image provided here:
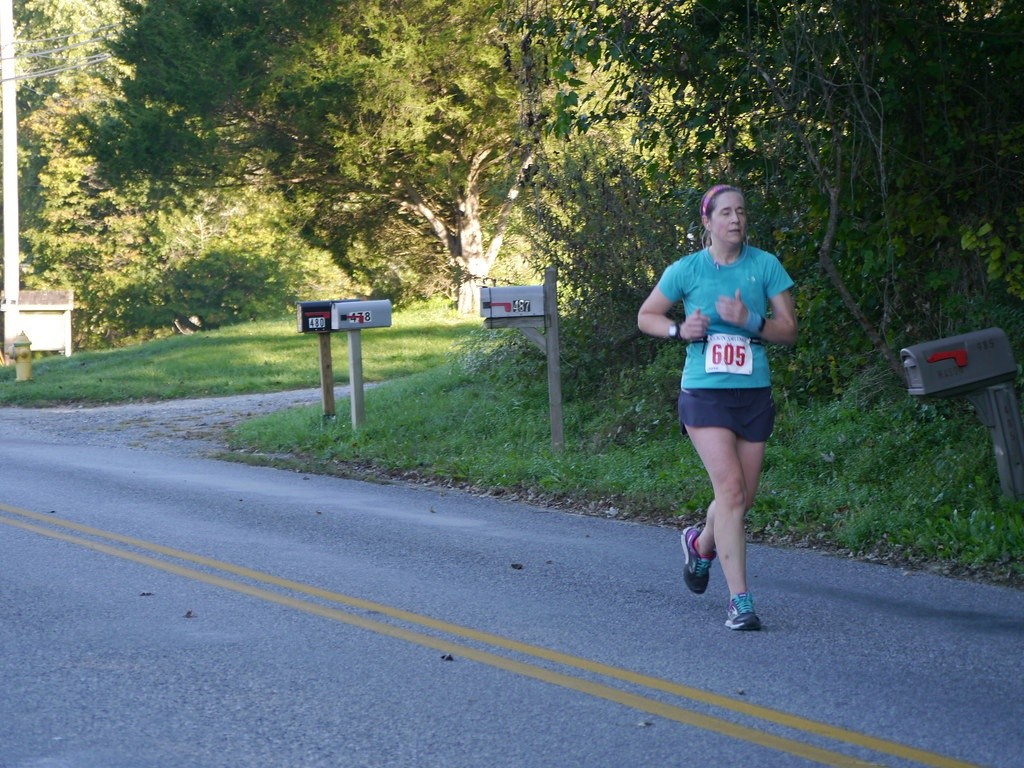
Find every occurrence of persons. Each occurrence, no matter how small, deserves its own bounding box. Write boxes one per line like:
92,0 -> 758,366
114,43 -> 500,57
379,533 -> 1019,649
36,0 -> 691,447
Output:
637,185 -> 801,629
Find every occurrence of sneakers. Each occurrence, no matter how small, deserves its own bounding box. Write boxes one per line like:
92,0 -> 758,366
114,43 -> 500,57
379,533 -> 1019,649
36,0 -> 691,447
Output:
724,592 -> 761,630
682,525 -> 718,595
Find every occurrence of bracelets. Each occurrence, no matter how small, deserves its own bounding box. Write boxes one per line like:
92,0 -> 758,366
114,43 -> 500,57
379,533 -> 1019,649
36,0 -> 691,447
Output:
757,317 -> 765,333
743,310 -> 761,333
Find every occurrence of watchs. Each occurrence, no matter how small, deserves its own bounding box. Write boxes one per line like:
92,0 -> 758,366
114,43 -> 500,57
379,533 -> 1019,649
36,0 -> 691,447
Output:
669,321 -> 684,340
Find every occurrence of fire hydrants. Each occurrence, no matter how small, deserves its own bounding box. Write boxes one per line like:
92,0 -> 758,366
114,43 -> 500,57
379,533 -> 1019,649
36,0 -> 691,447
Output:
7,331 -> 33,381
